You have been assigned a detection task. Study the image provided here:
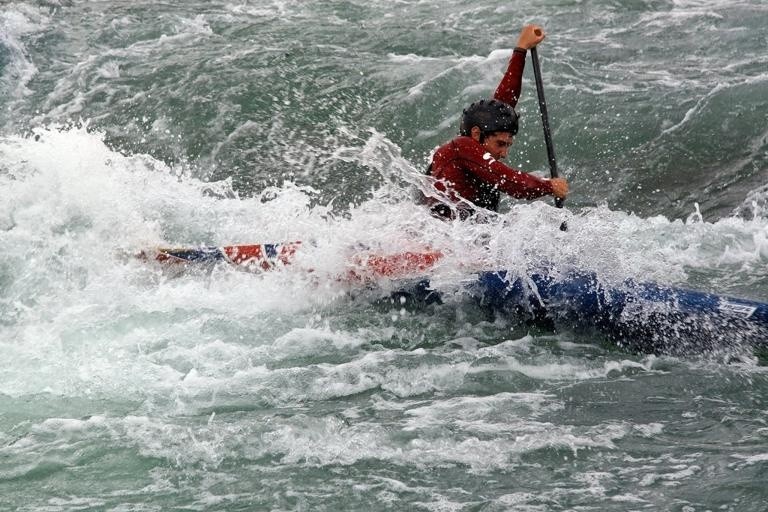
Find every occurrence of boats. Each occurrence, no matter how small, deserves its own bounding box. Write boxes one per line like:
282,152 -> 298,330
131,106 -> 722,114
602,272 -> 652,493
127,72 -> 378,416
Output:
121,240 -> 766,345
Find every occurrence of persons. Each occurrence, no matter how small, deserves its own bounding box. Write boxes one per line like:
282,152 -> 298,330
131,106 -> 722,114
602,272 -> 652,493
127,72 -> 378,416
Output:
413,24 -> 569,222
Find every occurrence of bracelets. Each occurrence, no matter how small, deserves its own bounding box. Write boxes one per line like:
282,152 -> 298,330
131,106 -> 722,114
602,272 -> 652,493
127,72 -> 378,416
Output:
512,46 -> 527,53
